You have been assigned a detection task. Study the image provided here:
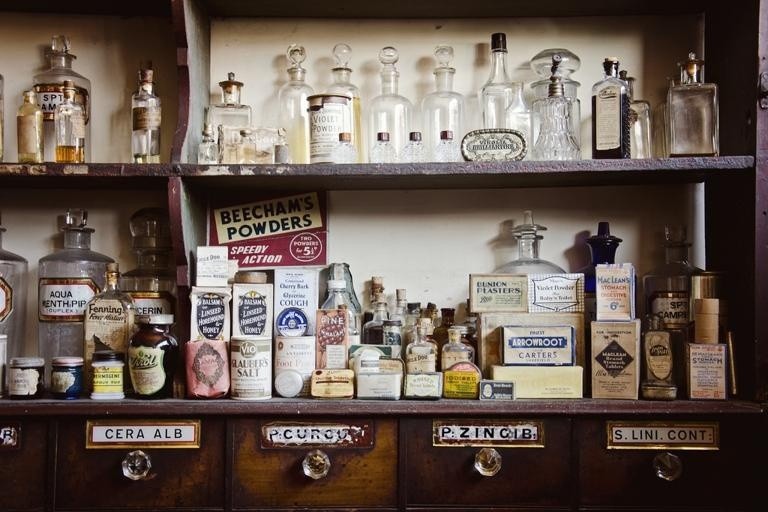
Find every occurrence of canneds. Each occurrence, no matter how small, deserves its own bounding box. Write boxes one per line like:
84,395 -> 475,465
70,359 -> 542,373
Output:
7,352 -> 128,401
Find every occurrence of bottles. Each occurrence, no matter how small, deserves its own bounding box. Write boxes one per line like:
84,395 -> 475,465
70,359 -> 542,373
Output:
198,70 -> 253,166
642,224 -> 705,398
55,80 -> 85,163
490,209 -> 566,273
130,67 -> 162,163
278,40 -> 468,164
574,220 -> 638,397
1,207 -> 174,399
17,91 -> 43,166
324,274 -> 480,401
31,32 -> 92,165
479,31 -> 722,155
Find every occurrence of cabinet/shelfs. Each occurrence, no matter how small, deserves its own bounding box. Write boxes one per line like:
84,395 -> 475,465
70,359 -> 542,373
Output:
0,1 -> 768,512
